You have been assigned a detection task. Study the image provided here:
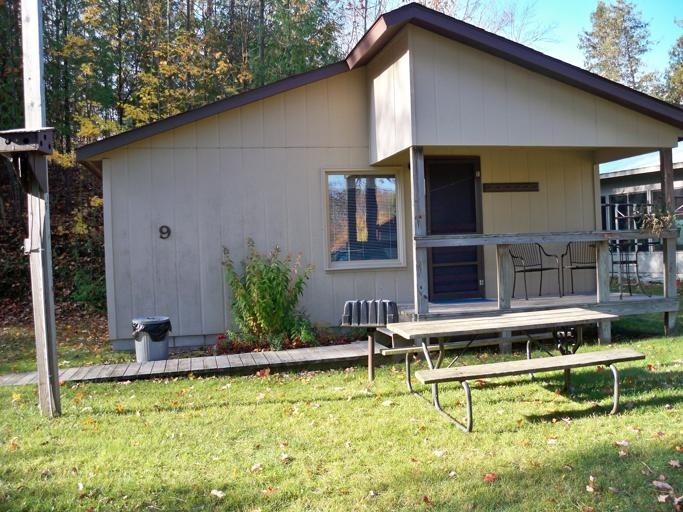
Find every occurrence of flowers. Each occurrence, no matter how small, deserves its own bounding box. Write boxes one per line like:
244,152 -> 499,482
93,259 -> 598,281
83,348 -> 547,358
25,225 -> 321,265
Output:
631,196 -> 677,238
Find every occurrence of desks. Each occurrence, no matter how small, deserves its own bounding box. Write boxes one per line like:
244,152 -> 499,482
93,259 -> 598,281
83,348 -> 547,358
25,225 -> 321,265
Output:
386,307 -> 619,430
589,242 -> 659,300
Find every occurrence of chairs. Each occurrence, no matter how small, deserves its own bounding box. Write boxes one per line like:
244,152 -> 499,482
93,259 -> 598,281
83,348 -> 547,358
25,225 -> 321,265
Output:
507,240 -> 595,301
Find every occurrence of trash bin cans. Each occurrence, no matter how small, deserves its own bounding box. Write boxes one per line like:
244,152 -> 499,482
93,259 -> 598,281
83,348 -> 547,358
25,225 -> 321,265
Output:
131,316 -> 171,363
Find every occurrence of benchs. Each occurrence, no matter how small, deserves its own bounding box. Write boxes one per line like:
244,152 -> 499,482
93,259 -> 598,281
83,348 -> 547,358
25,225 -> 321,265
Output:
379,332 -> 646,433
